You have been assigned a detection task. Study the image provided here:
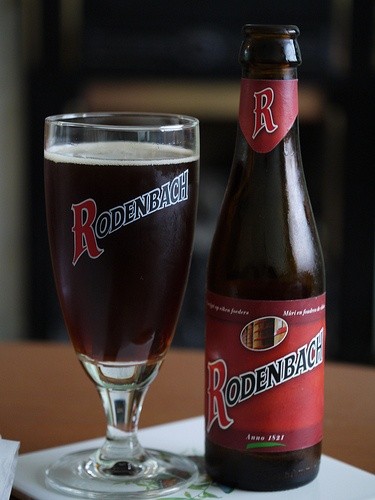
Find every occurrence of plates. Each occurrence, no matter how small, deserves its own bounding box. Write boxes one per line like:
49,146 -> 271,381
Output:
12,413 -> 374,496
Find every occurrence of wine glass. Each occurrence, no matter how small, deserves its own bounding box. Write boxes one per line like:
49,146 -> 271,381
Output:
42,111 -> 198,495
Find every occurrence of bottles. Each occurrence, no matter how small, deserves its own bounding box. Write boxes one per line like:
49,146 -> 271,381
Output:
204,23 -> 325,491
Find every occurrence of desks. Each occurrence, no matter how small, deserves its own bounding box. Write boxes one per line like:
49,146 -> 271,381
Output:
0,345 -> 375,500
